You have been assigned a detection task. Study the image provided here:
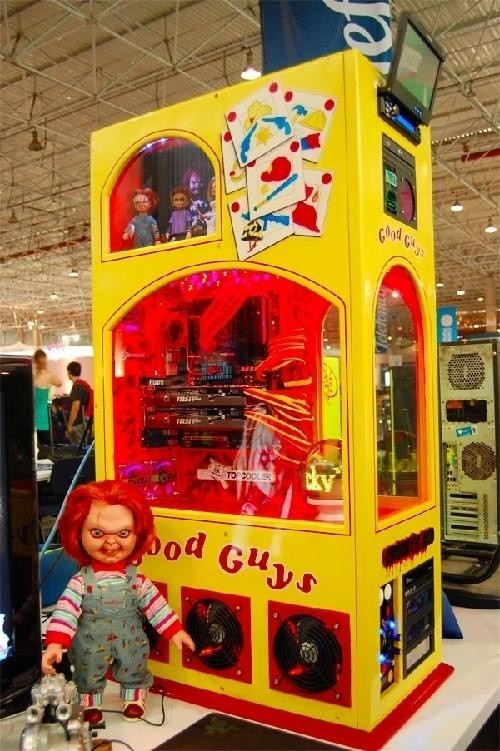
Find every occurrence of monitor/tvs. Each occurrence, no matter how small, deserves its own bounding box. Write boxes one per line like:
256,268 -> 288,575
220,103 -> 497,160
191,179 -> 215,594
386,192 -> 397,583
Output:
0,355 -> 43,721
389,11 -> 447,128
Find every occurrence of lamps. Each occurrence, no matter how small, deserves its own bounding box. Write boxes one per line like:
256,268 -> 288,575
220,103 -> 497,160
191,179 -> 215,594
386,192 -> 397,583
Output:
68,269 -> 79,277
436,271 -> 446,286
456,285 -> 465,296
29,130 -> 43,151
239,48 -> 262,80
475,292 -> 485,302
484,216 -> 497,233
451,199 -> 463,212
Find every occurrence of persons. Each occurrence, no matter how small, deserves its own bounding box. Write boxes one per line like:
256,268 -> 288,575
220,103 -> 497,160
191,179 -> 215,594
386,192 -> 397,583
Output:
41,478 -> 196,725
122,164 -> 216,250
30,348 -> 62,460
65,361 -> 94,443
